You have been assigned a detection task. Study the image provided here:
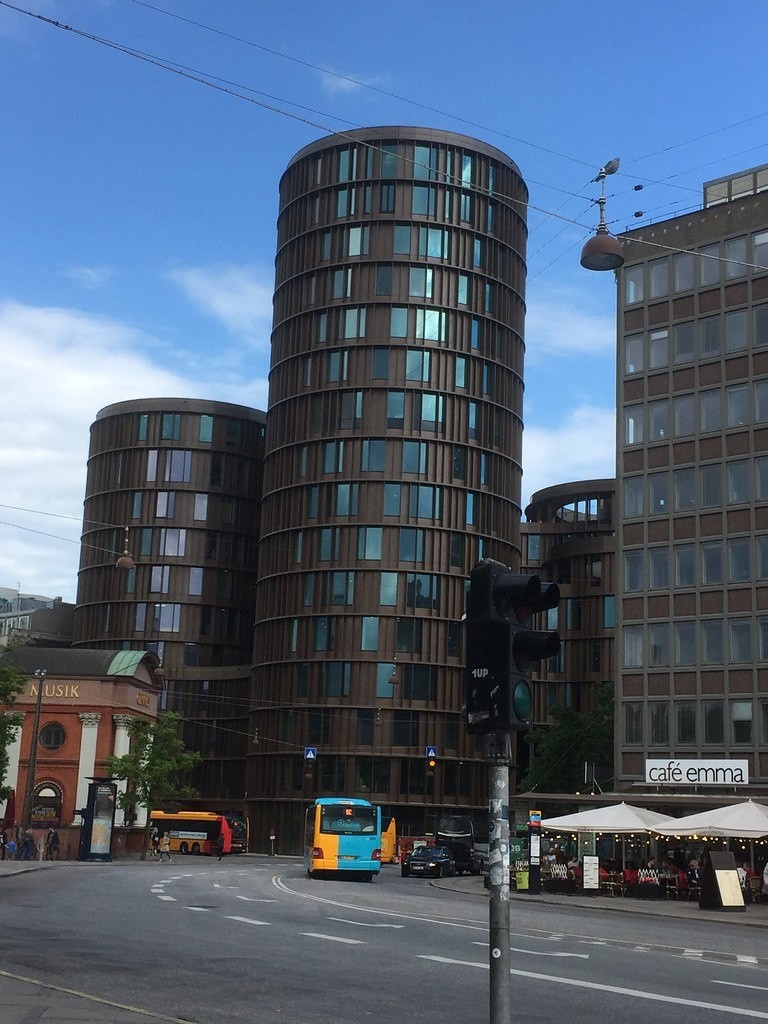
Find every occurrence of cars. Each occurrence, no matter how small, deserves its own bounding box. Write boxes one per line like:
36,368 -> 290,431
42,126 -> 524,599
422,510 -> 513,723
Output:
400,845 -> 457,879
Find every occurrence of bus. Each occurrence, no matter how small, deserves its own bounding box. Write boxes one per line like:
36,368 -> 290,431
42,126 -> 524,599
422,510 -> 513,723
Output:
304,797 -> 381,882
148,810 -> 249,856
436,814 -> 490,875
510,836 -> 574,864
380,816 -> 396,864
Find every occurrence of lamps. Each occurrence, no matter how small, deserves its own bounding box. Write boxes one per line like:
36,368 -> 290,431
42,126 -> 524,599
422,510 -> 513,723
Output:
389,667 -> 401,686
116,528 -> 136,569
303,768 -> 313,779
153,663 -> 165,676
579,177 -> 626,272
251,733 -> 259,744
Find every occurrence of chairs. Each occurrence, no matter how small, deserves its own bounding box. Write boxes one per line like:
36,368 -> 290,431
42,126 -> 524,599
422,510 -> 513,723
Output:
536,868 -> 767,906
324,820 -> 361,831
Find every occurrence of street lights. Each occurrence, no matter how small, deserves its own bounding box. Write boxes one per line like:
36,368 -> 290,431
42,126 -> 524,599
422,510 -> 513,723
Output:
21,669 -> 46,829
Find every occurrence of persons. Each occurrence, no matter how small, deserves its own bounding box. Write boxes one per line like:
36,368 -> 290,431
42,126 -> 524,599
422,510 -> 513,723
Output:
217,833 -> 225,861
0,827 -> 35,861
158,832 -> 172,862
151,827 -> 160,856
45,827 -> 58,861
550,844 -> 768,905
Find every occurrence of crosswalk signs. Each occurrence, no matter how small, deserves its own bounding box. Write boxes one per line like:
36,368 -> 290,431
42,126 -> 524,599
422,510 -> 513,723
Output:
305,748 -> 316,760
425,746 -> 436,758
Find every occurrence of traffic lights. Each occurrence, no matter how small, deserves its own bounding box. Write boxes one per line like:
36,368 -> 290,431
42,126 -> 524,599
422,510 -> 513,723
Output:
304,760 -> 313,776
463,557 -> 562,734
427,760 -> 436,770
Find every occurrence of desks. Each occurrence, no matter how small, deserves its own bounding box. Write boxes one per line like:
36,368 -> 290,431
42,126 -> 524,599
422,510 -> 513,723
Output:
598,873 -> 623,898
658,873 -> 679,900
542,870 -> 573,895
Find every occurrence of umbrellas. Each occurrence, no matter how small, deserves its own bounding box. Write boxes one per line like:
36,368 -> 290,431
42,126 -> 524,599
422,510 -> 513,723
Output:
528,798 -> 768,874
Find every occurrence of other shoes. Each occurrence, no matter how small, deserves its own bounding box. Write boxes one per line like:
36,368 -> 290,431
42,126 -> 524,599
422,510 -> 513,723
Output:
158,859 -> 162,863
168,858 -> 172,863
154,854 -> 156,856
150,854 -> 152,856
